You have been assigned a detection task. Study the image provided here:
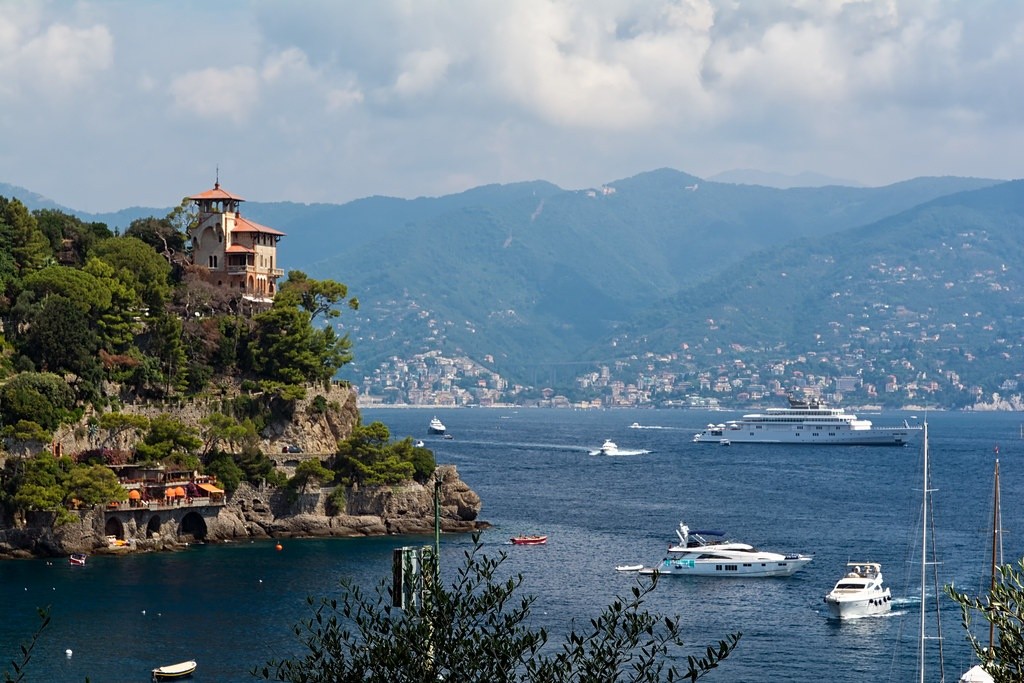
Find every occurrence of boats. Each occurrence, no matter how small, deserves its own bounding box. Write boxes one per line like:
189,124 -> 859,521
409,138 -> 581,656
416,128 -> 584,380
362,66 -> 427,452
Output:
510,532 -> 547,544
694,392 -> 922,446
639,521 -> 815,577
822,562 -> 892,620
615,564 -> 644,571
600,439 -> 618,452
68,552 -> 87,566
151,658 -> 197,683
427,415 -> 446,434
